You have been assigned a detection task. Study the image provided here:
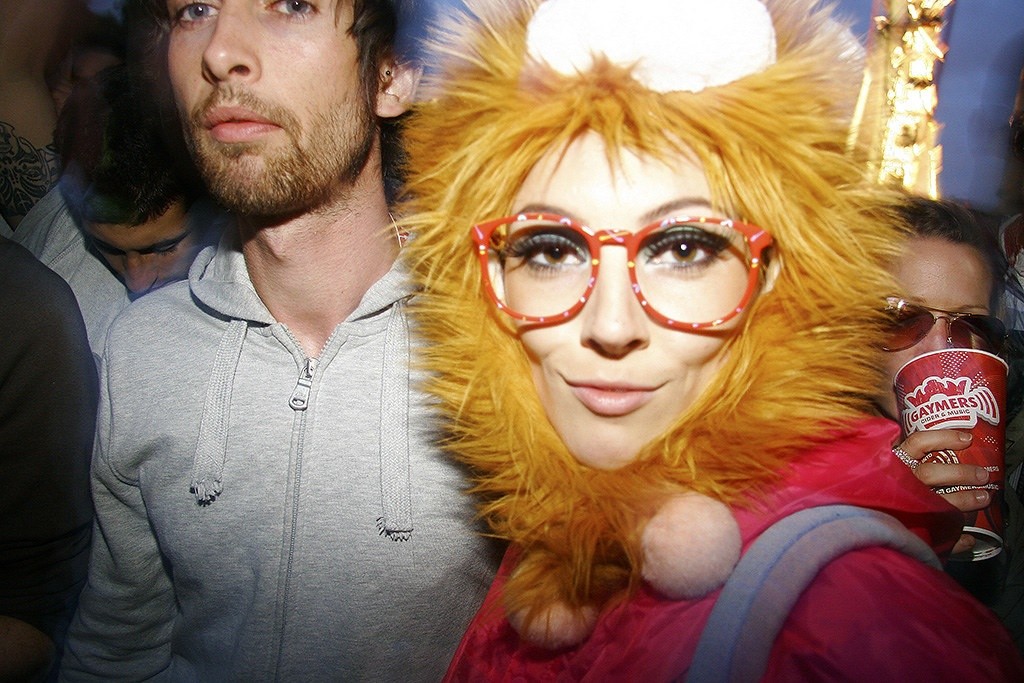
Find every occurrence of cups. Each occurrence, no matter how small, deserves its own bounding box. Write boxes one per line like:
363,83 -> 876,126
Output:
894,347 -> 1009,562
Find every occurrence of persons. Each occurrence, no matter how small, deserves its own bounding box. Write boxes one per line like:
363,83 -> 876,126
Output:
860,195 -> 1024,649
388,0 -> 1024,683
0,0 -> 231,683
62,0 -> 512,683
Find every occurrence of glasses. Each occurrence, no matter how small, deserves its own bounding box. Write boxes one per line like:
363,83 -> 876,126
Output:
468,213 -> 773,331
870,296 -> 1007,356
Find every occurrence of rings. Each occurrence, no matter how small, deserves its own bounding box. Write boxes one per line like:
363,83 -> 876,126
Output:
890,445 -> 918,469
